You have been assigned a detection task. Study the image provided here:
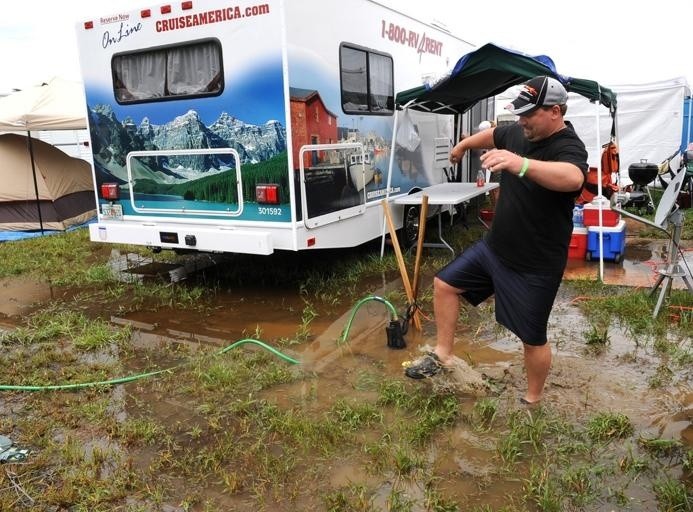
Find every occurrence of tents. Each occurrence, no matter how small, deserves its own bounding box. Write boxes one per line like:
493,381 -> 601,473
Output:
0,131 -> 97,233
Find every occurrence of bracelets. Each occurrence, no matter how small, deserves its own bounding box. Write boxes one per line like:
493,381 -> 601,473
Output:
515,155 -> 529,179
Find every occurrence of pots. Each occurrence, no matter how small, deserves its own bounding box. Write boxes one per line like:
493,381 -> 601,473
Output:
627,158 -> 659,186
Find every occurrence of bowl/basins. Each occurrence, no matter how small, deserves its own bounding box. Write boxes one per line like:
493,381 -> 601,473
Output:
479,207 -> 496,220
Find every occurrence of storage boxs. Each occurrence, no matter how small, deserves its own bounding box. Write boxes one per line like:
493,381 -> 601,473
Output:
566,203 -> 627,262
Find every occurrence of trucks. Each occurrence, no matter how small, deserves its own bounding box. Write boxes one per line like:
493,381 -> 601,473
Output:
70,0 -> 501,276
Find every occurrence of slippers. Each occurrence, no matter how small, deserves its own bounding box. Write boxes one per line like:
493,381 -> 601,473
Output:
404,351 -> 455,379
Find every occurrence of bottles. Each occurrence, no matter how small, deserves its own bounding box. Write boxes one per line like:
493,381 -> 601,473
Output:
572,204 -> 584,228
476,169 -> 485,188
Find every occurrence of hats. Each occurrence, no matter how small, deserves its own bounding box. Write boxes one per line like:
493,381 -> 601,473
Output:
503,75 -> 569,117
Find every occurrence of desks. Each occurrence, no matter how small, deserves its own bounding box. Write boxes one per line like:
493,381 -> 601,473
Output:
393,181 -> 499,260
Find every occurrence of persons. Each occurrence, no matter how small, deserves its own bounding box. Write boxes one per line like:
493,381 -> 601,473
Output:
403,75 -> 590,413
476,118 -> 503,211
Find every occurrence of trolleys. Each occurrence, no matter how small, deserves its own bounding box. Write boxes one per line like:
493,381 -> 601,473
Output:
615,189 -> 652,218
584,220 -> 628,264
